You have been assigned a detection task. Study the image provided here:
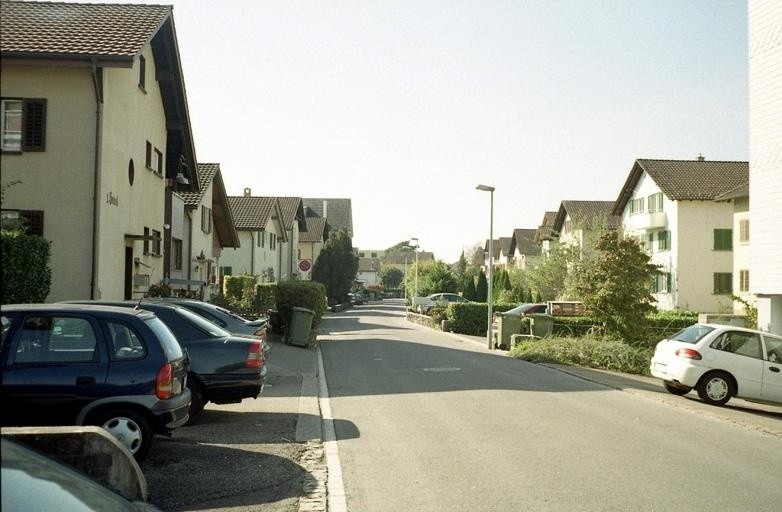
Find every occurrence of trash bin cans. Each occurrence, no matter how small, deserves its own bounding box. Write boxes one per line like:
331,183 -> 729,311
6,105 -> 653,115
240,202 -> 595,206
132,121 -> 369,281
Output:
286,307 -> 315,348
495,311 -> 525,350
526,313 -> 554,339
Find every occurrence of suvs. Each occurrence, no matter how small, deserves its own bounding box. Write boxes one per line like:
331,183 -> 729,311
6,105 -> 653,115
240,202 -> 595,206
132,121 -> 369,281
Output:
0,303 -> 192,463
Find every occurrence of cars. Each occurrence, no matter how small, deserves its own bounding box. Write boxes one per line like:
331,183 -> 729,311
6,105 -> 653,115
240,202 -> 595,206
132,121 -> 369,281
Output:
417,293 -> 474,315
54,299 -> 267,422
1,425 -> 160,512
649,323 -> 782,407
160,298 -> 270,365
490,303 -> 547,337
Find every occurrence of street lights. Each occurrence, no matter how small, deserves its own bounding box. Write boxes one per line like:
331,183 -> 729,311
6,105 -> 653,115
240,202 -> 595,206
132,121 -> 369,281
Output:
475,183 -> 495,351
411,238 -> 419,297
